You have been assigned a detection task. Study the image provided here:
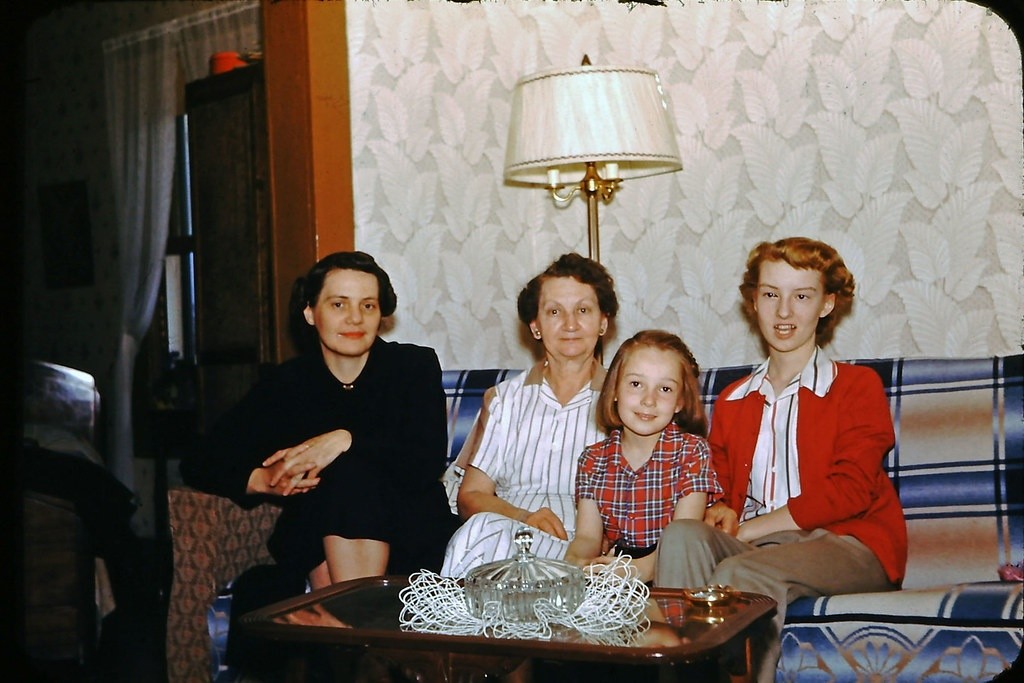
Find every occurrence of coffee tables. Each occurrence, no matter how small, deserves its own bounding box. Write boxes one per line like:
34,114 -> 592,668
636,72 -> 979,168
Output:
231,574 -> 778,683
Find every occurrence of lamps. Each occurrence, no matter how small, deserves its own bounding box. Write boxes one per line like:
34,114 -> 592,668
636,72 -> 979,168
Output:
501,55 -> 684,263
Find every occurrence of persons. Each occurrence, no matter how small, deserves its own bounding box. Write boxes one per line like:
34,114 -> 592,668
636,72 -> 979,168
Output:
564,329 -> 724,586
176,251 -> 449,591
440,251 -> 618,579
653,236 -> 907,683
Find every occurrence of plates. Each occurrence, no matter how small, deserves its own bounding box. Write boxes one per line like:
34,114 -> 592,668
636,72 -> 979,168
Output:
684,585 -> 739,606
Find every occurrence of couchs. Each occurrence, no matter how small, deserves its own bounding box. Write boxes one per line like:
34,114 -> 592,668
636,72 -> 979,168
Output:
203,354 -> 1024,683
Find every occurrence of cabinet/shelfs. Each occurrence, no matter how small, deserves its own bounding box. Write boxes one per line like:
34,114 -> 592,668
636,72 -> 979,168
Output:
187,63 -> 274,360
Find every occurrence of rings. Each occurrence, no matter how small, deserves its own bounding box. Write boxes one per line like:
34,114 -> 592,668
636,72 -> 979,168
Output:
601,551 -> 606,555
304,443 -> 308,450
